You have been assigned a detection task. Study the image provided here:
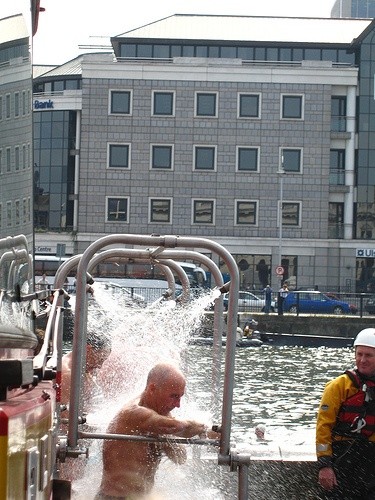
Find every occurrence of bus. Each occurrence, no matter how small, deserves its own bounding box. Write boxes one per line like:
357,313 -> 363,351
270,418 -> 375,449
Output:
18,255 -> 207,304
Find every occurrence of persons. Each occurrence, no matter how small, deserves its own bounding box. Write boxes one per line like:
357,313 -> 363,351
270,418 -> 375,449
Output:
278,284 -> 289,314
93,362 -> 223,500
316,328 -> 375,500
37,273 -> 49,283
96,324 -> 182,403
260,284 -> 274,313
254,424 -> 266,440
236,323 -> 243,342
52,332 -> 111,430
244,322 -> 260,338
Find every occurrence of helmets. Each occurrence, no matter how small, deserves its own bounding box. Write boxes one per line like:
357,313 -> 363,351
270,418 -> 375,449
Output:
353,328 -> 375,348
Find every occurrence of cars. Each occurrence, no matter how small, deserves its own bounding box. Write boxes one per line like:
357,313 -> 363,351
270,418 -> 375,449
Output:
283,291 -> 350,315
222,290 -> 275,312
322,293 -> 358,314
365,295 -> 375,314
252,295 -> 276,308
